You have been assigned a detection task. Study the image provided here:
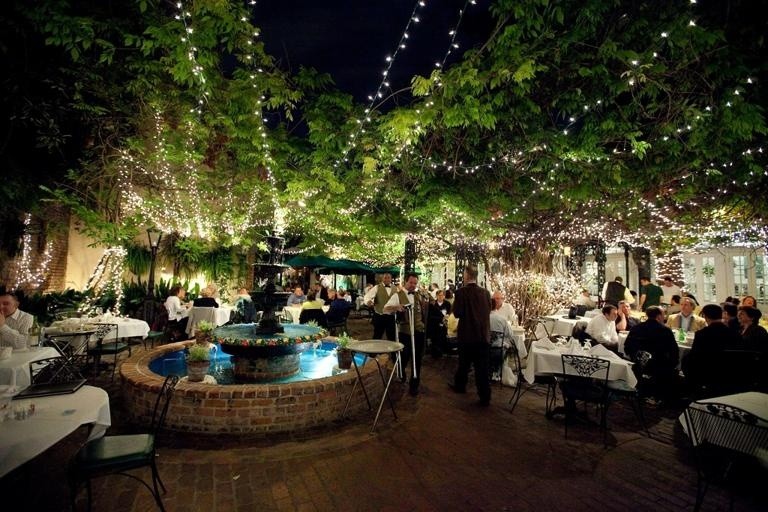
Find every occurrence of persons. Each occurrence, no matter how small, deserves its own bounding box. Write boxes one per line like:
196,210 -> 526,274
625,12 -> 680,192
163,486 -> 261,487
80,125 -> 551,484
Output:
1,292 -> 33,350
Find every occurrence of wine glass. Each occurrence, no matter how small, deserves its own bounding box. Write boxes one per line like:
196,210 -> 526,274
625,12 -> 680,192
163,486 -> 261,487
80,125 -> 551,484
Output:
556,335 -> 593,354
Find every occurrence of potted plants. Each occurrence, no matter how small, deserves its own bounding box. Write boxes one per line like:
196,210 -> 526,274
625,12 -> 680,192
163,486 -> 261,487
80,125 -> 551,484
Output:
336,331 -> 356,369
186,344 -> 212,382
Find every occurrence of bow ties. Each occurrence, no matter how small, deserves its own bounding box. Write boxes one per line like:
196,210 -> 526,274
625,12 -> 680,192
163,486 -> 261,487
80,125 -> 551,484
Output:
408,291 -> 415,295
385,284 -> 391,288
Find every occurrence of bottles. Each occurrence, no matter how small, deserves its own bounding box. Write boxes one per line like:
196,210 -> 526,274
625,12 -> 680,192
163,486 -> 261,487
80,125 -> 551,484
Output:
28,313 -> 42,349
679,327 -> 686,342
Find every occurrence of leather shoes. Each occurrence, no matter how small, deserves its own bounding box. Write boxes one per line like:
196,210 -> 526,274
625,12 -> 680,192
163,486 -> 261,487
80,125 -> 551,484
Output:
396,369 -> 501,407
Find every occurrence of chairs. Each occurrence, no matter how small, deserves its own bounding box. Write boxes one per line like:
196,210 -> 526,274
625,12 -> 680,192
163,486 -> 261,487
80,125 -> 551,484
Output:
1,307 -> 183,512
609,350 -> 653,434
559,354 -> 611,448
507,339 -> 558,419
682,399 -> 767,511
440,294 -> 768,398
143,281 -> 440,347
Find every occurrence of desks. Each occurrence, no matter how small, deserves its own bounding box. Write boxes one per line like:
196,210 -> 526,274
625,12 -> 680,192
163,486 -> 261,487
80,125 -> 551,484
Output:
524,338 -> 636,427
340,338 -> 405,436
679,392 -> 768,482
